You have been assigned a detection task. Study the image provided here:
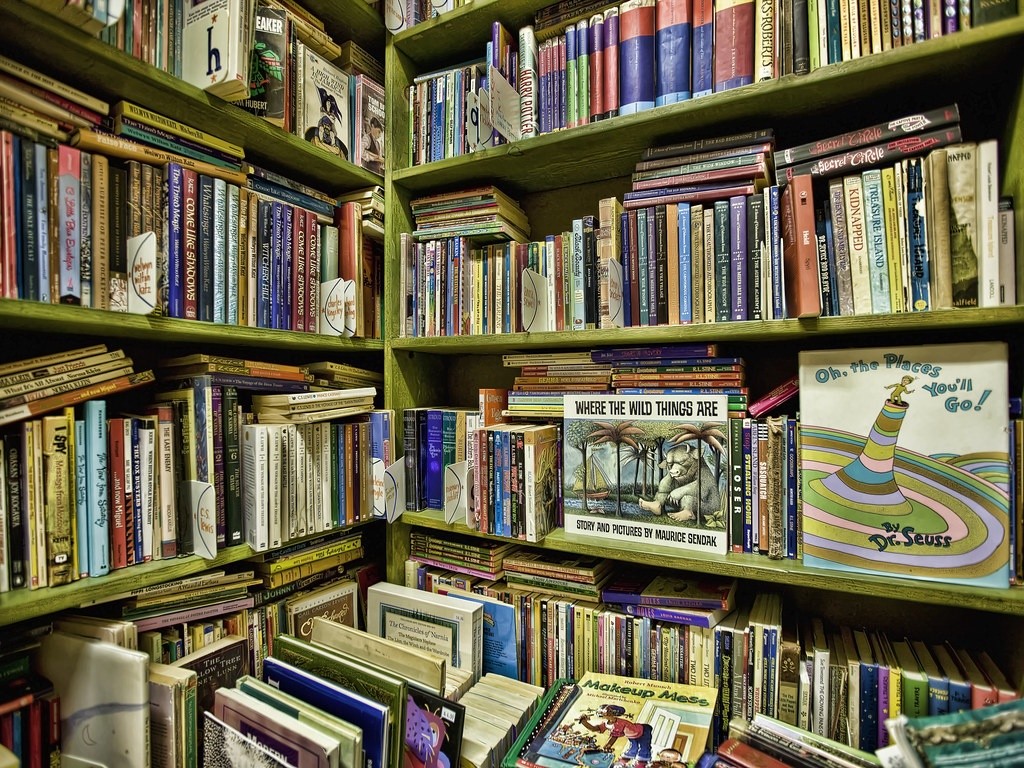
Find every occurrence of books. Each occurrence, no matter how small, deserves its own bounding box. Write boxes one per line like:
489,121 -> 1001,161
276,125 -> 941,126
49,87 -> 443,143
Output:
391,0 -> 1024,338
0,340 -> 1024,768
0,0 -> 385,341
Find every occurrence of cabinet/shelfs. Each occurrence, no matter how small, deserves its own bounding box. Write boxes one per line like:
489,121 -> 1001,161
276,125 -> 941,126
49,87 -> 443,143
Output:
0,0 -> 1024,768
